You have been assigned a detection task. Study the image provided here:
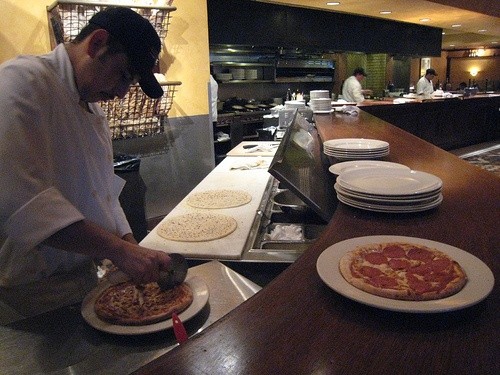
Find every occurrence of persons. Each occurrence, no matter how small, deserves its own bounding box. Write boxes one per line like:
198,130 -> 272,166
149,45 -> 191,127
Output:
210,74 -> 218,140
1,8 -> 173,327
342,68 -> 372,102
416,68 -> 438,95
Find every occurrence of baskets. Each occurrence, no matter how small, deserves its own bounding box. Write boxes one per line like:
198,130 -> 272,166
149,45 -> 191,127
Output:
98,82 -> 182,141
46,0 -> 177,72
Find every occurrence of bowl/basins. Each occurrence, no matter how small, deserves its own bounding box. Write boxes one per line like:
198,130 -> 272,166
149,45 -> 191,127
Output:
273,97 -> 283,104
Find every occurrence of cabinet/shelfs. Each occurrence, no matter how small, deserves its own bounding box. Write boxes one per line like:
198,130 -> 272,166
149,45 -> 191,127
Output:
210,61 -> 274,84
46,0 -> 181,140
401,109 -> 449,149
453,108 -> 482,147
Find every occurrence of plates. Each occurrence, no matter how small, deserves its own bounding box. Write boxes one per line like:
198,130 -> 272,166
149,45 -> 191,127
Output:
79,266 -> 209,337
334,167 -> 443,213
322,137 -> 391,159
245,105 -> 257,109
216,70 -> 232,86
234,68 -> 245,82
309,90 -> 333,115
329,160 -> 412,176
316,234 -> 496,315
232,105 -> 244,110
247,68 -> 259,83
284,101 -> 307,109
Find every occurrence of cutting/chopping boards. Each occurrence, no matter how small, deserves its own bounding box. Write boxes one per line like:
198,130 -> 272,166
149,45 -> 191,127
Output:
138,156 -> 273,261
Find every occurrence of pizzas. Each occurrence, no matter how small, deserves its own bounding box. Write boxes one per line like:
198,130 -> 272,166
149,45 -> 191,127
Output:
93,277 -> 195,325
338,241 -> 466,302
187,189 -> 252,209
157,210 -> 238,242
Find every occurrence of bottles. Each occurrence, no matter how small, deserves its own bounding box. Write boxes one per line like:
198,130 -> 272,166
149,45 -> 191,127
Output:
445,76 -> 450,92
285,87 -> 293,101
296,88 -> 301,102
436,78 -> 442,90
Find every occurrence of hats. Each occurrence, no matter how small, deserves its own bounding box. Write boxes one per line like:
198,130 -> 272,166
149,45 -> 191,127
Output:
354,67 -> 368,76
88,7 -> 163,99
426,69 -> 438,76
